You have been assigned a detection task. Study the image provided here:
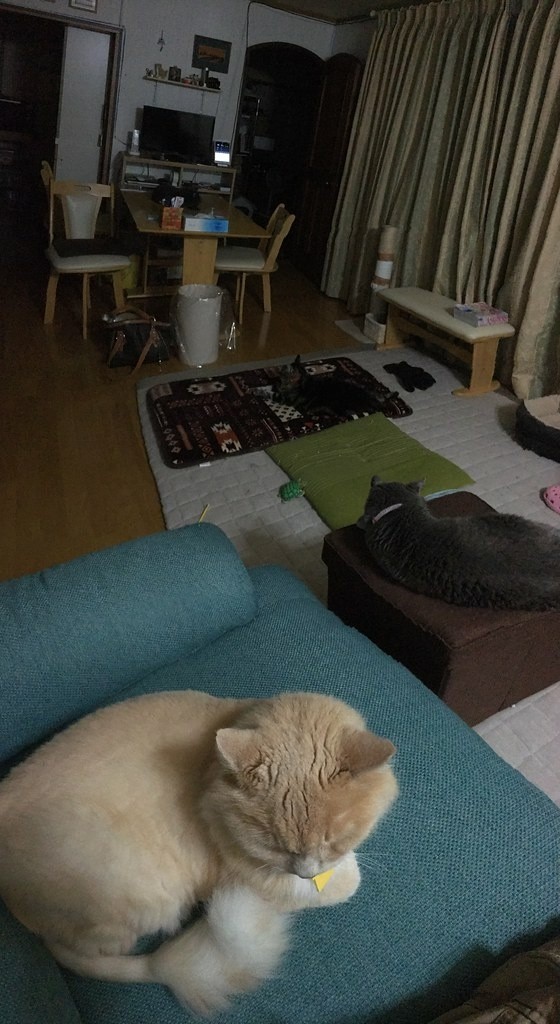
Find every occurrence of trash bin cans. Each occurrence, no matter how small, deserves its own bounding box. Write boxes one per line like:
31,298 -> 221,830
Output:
178,284 -> 223,366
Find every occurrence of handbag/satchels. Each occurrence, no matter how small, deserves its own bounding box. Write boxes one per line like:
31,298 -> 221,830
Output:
94,304 -> 177,378
152,179 -> 201,208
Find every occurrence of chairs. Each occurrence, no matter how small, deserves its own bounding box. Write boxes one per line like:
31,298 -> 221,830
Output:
213,203 -> 296,325
39,160 -> 119,308
43,179 -> 131,339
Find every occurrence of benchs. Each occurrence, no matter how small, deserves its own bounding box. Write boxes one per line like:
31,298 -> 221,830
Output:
375,287 -> 515,397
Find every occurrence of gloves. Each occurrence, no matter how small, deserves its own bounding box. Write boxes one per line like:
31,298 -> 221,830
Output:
383,361 -> 436,392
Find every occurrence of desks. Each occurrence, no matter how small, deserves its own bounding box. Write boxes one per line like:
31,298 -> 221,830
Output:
120,189 -> 273,341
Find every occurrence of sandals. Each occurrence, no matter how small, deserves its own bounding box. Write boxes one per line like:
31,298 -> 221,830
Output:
543,482 -> 560,514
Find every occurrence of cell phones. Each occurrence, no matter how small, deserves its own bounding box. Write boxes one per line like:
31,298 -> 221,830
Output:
214,140 -> 231,166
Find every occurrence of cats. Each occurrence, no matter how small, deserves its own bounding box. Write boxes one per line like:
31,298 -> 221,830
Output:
1,688 -> 400,1019
363,477 -> 560,613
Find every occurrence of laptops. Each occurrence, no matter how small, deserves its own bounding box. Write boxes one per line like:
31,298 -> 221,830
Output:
139,106 -> 216,167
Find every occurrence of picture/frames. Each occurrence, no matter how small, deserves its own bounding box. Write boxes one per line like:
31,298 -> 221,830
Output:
68,0 -> 98,14
191,35 -> 232,74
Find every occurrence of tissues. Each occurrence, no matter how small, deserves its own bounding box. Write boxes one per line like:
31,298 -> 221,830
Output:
182,206 -> 229,233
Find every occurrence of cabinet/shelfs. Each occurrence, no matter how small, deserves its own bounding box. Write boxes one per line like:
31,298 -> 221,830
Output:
115,150 -> 237,246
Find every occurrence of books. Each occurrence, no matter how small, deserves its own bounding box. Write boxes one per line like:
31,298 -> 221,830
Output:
454,301 -> 508,327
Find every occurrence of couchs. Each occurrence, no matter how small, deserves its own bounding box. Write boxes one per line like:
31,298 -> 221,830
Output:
0,523 -> 560,1024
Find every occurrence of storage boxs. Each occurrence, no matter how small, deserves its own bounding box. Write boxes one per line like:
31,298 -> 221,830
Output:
453,301 -> 508,328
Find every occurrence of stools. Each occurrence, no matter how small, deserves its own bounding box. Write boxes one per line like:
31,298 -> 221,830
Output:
321,490 -> 560,729
